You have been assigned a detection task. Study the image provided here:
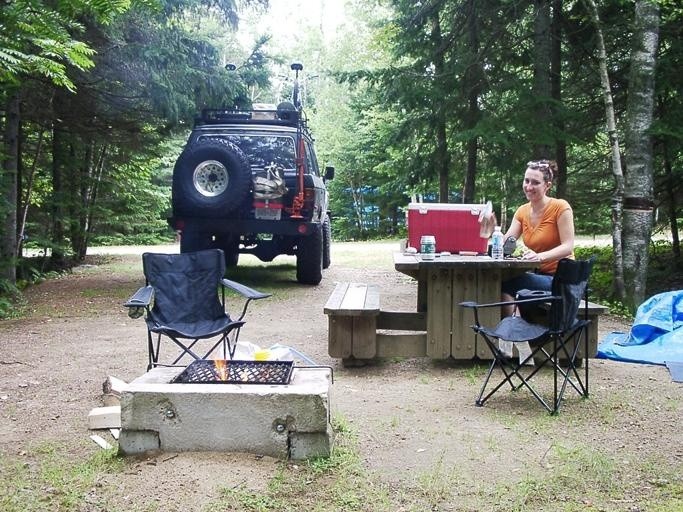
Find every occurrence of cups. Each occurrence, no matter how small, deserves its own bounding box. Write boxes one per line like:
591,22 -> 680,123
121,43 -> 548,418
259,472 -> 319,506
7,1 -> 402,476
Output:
486,245 -> 493,257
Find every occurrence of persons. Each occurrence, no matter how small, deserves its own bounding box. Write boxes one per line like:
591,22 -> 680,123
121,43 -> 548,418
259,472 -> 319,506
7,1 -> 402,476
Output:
501,159 -> 576,319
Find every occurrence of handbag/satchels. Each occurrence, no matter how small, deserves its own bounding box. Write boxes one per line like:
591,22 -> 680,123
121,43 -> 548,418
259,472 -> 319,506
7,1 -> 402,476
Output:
517,287 -> 552,298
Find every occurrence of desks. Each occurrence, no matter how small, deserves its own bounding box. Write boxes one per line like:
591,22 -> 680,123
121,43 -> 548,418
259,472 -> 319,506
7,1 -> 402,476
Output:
394,251 -> 541,360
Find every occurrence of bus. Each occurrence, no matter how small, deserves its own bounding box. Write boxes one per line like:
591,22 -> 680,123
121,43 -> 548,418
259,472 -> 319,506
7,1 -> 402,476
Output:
341,185 -> 462,239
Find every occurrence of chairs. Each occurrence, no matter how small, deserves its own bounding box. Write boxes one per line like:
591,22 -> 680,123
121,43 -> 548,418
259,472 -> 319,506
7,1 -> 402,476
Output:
458,257 -> 596,414
123,249 -> 272,372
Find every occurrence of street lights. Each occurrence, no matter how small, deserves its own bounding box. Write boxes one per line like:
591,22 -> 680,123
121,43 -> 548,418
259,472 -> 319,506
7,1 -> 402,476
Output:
289,60 -> 302,107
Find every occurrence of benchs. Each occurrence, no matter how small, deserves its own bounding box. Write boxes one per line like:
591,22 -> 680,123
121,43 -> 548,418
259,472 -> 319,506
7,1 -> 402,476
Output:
324,282 -> 380,359
538,300 -> 609,359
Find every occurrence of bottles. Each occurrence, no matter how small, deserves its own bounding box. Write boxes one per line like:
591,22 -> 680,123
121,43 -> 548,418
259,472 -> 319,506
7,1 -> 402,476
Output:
419,235 -> 436,261
490,226 -> 505,260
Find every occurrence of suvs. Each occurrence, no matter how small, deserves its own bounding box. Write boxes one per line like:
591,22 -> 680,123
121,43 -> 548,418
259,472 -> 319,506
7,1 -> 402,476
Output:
165,101 -> 334,285
